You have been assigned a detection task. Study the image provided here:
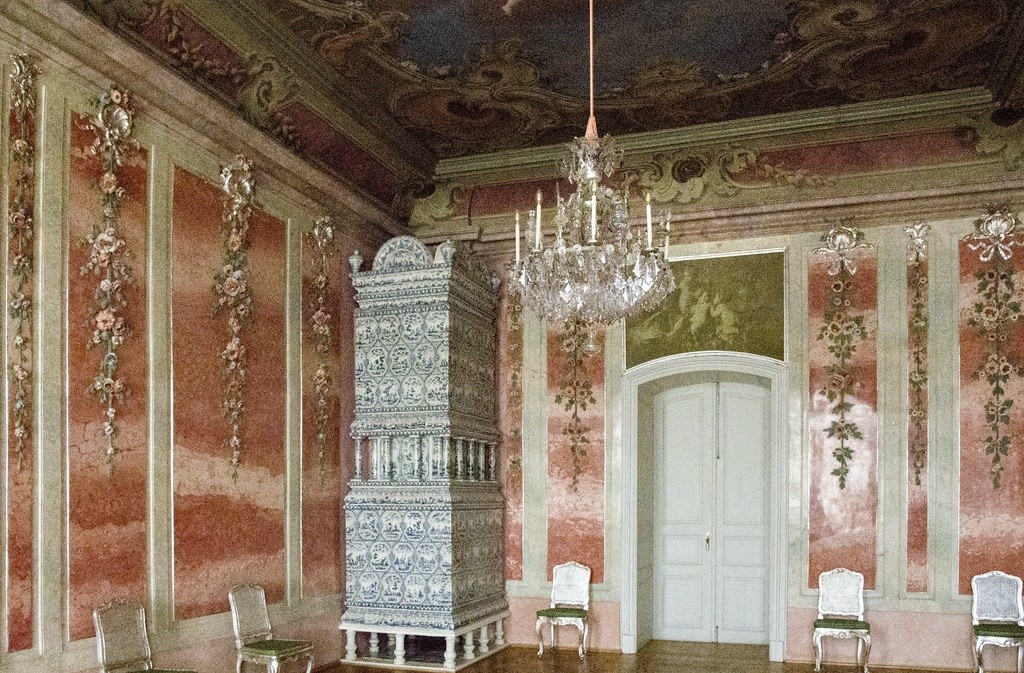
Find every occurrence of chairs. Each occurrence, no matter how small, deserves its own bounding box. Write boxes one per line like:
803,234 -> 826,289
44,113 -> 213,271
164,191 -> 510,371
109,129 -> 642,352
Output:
812,567 -> 871,673
229,583 -> 315,673
971,571 -> 1024,673
535,561 -> 592,661
92,597 -> 198,673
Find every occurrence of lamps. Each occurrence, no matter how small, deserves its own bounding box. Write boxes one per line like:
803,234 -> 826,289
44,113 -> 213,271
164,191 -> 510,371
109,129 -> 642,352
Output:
506,1 -> 675,326
